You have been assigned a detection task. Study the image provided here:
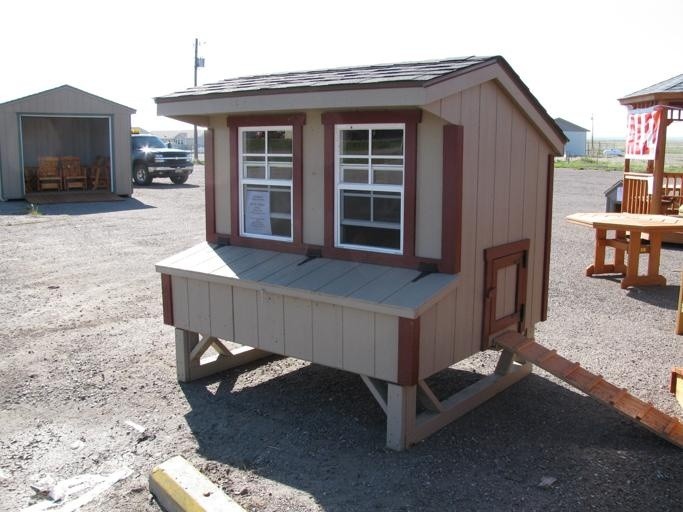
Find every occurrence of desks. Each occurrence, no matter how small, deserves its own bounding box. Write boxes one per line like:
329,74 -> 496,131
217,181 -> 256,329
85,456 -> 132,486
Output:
566,213 -> 683,289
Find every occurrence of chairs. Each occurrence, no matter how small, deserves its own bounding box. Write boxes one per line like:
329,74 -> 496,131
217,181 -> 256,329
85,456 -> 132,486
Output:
24,155 -> 110,192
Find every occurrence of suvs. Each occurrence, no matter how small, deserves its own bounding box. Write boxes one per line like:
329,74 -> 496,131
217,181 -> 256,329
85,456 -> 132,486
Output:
131,134 -> 194,187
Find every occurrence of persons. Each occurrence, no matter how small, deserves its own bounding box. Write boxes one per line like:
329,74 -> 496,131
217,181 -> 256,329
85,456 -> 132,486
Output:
166,140 -> 172,148
